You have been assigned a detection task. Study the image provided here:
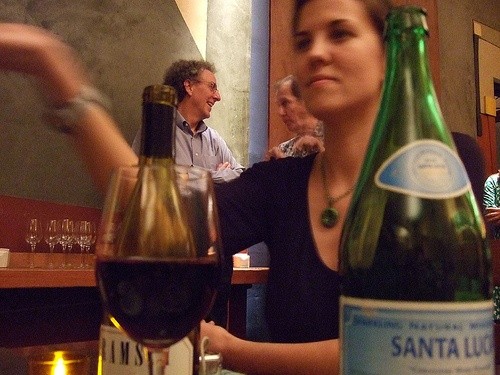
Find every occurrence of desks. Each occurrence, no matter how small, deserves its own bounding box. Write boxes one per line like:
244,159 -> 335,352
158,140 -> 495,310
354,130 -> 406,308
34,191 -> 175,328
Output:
0,267 -> 270,375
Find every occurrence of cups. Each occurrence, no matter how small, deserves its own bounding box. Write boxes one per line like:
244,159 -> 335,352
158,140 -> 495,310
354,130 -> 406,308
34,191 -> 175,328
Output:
0,248 -> 11,267
25,350 -> 91,375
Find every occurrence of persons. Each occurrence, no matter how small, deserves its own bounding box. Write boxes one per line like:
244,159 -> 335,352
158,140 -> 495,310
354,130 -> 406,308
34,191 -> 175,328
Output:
0,0 -> 494,375
482,171 -> 500,364
132,59 -> 248,331
263,74 -> 325,158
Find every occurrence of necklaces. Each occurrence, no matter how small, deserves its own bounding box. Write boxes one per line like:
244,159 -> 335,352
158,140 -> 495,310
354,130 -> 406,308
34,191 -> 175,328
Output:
318,160 -> 356,226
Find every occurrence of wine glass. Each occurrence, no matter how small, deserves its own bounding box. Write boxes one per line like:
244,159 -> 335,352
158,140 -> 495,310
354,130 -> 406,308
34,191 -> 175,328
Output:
23,218 -> 43,269
96,165 -> 222,375
43,220 -> 62,269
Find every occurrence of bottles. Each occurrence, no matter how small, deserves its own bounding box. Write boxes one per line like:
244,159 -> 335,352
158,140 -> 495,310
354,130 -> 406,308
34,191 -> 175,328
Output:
337,6 -> 498,375
57,219 -> 97,270
98,83 -> 200,375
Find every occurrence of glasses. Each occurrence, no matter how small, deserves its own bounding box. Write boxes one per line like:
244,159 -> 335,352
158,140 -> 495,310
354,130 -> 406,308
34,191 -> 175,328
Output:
201,80 -> 217,94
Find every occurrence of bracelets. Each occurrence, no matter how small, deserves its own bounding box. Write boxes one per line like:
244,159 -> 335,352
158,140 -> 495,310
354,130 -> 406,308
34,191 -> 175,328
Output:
42,88 -> 113,133
